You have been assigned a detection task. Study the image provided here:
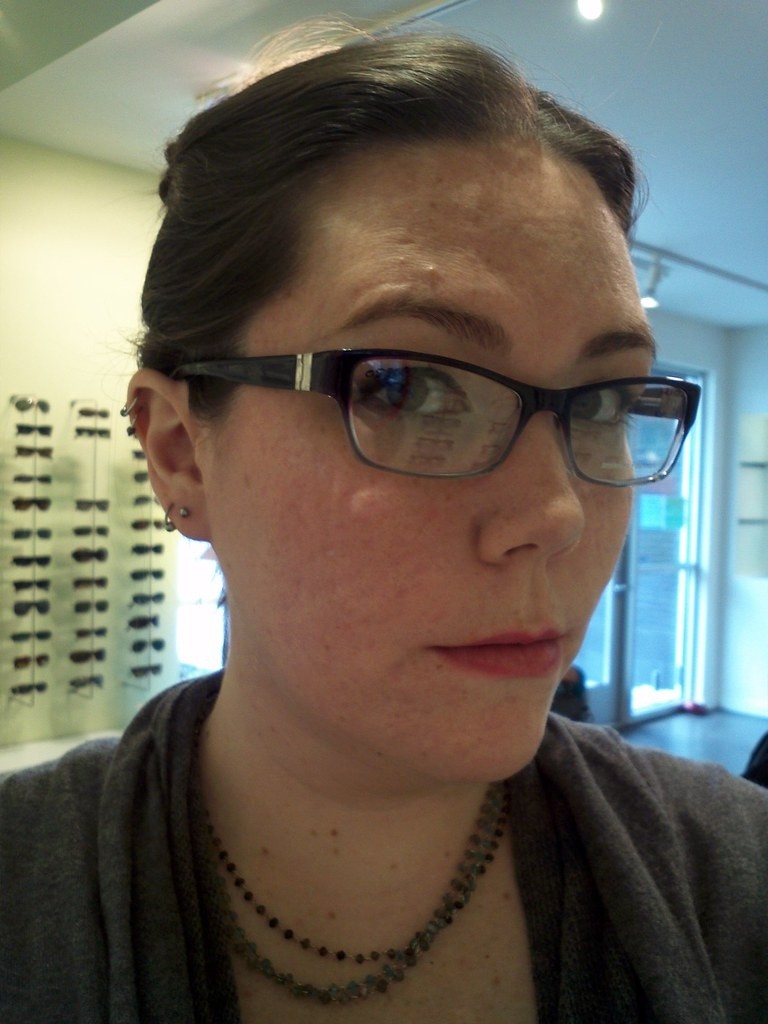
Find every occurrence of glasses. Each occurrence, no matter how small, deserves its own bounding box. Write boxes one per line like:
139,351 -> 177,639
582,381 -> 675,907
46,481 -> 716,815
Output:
170,348 -> 700,488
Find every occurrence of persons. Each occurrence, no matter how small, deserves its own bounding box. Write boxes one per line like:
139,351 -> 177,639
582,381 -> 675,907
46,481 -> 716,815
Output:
0,28 -> 768,1023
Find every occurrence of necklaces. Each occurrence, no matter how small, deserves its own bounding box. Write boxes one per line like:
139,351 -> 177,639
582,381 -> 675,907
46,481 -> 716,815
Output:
185,780 -> 513,1001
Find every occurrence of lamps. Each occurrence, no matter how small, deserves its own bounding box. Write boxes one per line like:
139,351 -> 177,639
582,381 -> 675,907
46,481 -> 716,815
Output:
643,264 -> 668,308
